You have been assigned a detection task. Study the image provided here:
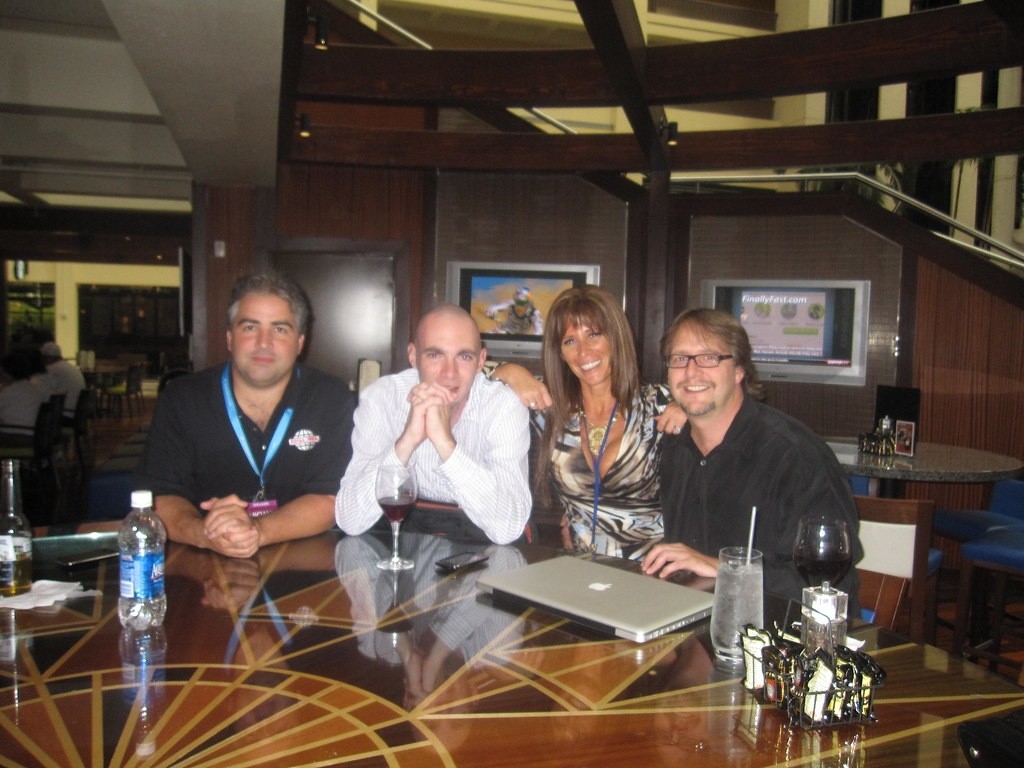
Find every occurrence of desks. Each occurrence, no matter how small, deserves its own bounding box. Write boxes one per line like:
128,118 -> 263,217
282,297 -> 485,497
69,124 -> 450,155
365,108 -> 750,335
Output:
814,443 -> 1018,642
1,502 -> 1023,767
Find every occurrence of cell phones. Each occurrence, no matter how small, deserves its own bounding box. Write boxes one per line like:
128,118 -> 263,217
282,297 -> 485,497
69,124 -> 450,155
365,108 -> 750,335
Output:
53,547 -> 120,567
435,552 -> 489,570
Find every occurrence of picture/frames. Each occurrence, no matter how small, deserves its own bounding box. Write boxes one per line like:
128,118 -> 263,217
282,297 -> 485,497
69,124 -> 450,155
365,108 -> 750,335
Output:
893,421 -> 916,457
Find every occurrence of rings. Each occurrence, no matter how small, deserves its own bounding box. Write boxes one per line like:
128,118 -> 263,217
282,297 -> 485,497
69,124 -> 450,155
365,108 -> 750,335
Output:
674,427 -> 681,434
530,401 -> 538,406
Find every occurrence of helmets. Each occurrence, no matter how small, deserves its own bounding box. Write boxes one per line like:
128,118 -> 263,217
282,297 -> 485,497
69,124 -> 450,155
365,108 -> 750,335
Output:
513,288 -> 533,319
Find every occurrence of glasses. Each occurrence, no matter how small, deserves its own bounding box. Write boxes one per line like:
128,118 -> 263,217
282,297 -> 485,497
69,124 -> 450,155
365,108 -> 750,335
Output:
666,353 -> 733,368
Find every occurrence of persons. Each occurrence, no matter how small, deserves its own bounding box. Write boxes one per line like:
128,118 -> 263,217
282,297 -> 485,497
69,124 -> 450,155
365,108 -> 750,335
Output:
0,340 -> 87,446
560,306 -> 865,620
481,285 -> 689,562
334,531 -> 531,755
525,579 -> 735,768
164,531 -> 338,765
137,272 -> 358,560
334,303 -> 534,545
484,287 -> 544,336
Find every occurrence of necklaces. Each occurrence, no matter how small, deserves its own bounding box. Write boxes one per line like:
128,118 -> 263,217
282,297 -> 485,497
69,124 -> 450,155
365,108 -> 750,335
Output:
580,397 -> 622,552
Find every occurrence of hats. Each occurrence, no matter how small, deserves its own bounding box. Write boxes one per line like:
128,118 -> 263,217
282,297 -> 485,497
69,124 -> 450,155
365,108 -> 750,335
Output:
40,342 -> 60,357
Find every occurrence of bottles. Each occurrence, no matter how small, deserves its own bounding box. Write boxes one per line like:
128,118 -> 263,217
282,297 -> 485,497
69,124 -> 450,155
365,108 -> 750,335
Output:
802,579 -> 849,655
0,610 -> 33,726
119,628 -> 168,757
0,459 -> 33,597
117,489 -> 167,630
875,418 -> 894,456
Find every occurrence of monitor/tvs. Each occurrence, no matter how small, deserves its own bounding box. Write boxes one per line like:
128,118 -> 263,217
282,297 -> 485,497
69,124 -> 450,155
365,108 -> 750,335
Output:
461,269 -> 587,342
178,247 -> 192,337
715,287 -> 855,367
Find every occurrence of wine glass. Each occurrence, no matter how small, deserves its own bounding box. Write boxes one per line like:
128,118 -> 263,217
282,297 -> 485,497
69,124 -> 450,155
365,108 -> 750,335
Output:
374,464 -> 418,570
372,572 -> 417,665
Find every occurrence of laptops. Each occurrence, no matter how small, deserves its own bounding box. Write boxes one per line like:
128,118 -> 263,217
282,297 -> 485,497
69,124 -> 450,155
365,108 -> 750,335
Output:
475,555 -> 715,643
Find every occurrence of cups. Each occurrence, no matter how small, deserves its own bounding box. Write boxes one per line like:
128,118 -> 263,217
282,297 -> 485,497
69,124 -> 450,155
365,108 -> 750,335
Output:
792,516 -> 851,589
709,546 -> 764,662
858,431 -> 878,453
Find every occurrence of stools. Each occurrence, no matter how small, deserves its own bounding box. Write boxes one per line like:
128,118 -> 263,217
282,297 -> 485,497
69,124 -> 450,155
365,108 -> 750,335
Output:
849,434 -> 1024,684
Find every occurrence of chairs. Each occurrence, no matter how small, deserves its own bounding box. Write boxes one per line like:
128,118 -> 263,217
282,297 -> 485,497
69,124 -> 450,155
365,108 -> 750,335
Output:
0,350 -> 191,514
